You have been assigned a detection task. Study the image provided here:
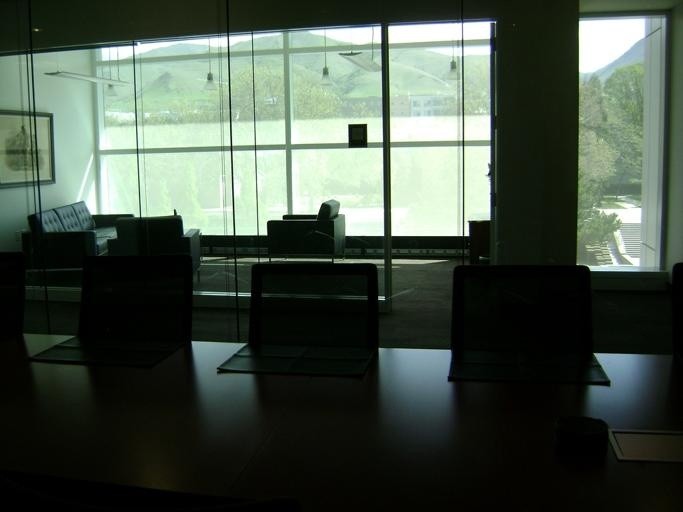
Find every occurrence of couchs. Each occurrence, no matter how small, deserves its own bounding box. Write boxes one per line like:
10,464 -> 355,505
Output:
19,200 -> 133,256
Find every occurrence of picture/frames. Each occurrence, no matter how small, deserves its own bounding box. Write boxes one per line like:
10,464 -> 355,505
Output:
0,109 -> 54,190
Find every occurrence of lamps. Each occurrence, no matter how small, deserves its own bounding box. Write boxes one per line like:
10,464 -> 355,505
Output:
44,45 -> 131,89
445,22 -> 461,82
202,38 -> 219,92
319,29 -> 333,86
337,26 -> 382,73
104,46 -> 118,99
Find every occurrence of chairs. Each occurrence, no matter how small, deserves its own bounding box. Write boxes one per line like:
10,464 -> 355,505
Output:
246,262 -> 380,348
76,256 -> 192,338
466,221 -> 492,263
265,200 -> 344,258
106,215 -> 201,282
450,264 -> 594,347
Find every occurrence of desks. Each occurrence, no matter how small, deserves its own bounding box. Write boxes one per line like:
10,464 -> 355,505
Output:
0,332 -> 681,512
